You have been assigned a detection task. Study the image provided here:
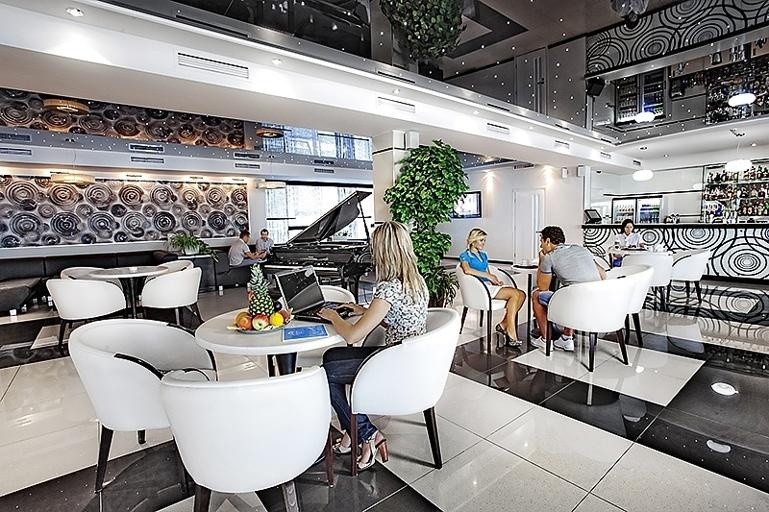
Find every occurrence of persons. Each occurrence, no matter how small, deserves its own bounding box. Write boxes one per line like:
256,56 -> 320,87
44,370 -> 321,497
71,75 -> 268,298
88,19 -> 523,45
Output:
530,226 -> 602,352
613,219 -> 645,250
255,228 -> 274,254
316,221 -> 430,472
459,228 -> 526,347
228,231 -> 260,268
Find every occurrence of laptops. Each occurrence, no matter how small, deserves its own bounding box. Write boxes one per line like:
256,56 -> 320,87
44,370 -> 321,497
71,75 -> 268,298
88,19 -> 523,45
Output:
272,264 -> 356,325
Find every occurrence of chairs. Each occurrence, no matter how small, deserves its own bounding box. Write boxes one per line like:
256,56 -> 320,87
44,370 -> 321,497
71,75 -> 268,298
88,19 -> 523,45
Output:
456,240 -> 710,371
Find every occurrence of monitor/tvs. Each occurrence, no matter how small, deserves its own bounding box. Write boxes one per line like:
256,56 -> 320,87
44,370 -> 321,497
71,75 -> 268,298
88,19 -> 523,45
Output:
584,209 -> 602,224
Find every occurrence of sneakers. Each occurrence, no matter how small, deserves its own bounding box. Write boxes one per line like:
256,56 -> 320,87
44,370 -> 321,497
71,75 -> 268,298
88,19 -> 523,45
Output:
554,337 -> 574,351
530,335 -> 554,353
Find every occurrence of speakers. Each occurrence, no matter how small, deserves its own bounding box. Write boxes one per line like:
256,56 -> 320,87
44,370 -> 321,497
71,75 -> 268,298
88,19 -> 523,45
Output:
587,77 -> 606,98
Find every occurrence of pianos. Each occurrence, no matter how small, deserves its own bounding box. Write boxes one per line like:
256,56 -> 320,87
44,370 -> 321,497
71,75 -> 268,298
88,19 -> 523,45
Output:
263,192 -> 374,306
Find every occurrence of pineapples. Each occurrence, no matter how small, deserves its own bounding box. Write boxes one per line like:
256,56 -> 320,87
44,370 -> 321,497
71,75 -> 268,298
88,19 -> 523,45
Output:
248,263 -> 275,317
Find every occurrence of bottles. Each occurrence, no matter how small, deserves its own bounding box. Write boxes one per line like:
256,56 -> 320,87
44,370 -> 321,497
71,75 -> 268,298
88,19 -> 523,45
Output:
701,165 -> 769,223
617,45 -> 768,123
613,203 -> 661,223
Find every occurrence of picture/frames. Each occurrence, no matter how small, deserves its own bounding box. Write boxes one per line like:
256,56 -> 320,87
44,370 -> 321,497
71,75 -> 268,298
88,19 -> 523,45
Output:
451,189 -> 483,220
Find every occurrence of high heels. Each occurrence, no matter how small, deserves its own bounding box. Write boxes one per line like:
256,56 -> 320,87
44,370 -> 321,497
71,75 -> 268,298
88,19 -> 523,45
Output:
356,430 -> 388,471
503,328 -> 523,346
333,433 -> 352,454
496,324 -> 506,347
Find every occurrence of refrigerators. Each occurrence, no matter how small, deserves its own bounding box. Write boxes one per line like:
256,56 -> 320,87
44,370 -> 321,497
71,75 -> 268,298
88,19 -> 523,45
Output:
247,188 -> 288,246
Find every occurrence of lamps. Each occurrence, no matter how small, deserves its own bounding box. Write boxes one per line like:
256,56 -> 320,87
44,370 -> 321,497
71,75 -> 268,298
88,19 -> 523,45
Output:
50,149 -> 96,185
257,157 -> 286,189
254,123 -> 284,139
42,96 -> 89,114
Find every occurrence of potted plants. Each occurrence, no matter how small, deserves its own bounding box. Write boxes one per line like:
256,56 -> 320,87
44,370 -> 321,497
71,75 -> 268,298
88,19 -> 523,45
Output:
163,231 -> 214,256
382,139 -> 469,309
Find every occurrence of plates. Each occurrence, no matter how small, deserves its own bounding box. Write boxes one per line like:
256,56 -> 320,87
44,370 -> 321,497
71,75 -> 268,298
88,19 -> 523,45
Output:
236,324 -> 288,334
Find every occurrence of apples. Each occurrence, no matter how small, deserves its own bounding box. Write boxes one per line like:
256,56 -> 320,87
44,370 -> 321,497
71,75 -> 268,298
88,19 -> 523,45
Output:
236,312 -> 252,330
252,315 -> 269,331
278,310 -> 288,323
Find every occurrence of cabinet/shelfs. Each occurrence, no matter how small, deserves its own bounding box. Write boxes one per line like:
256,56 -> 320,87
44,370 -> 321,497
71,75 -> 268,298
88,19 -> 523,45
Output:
699,160 -> 769,223
